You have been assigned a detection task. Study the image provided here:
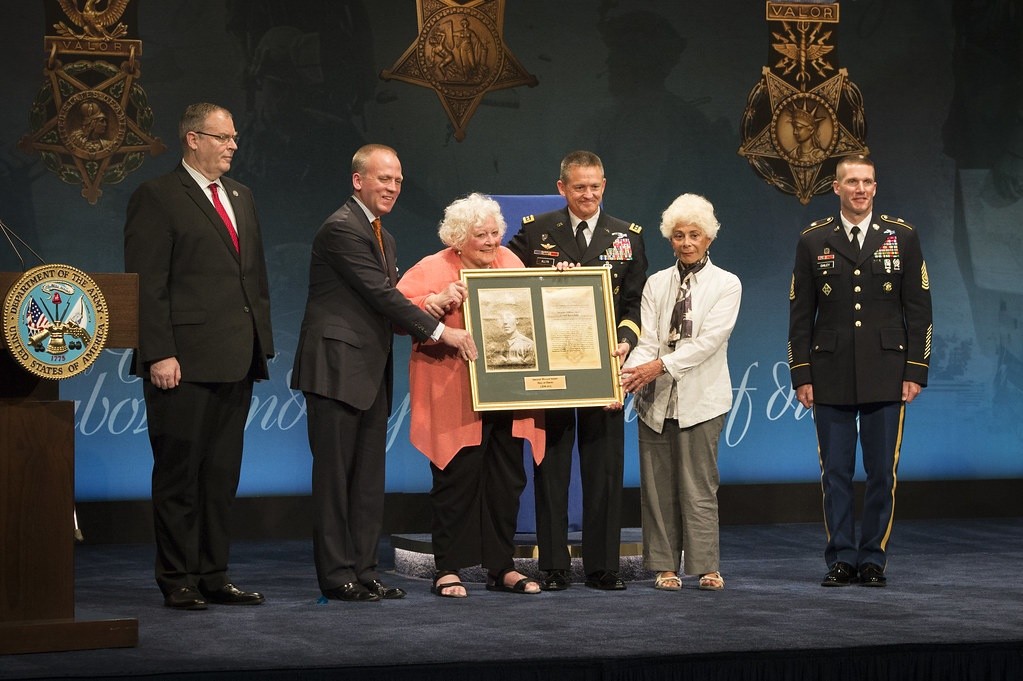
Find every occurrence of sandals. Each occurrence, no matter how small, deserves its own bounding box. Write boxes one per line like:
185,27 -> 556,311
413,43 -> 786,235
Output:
486,568 -> 541,594
698,571 -> 724,590
654,571 -> 682,591
431,570 -> 467,598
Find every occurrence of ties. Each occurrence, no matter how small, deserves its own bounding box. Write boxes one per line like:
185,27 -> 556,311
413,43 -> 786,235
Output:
502,342 -> 509,361
850,226 -> 861,254
208,183 -> 240,257
370,218 -> 388,272
575,221 -> 588,258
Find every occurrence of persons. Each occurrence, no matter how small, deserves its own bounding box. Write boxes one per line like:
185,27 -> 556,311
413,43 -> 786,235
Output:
289,145 -> 478,603
394,192 -> 547,597
122,103 -> 275,609
603,193 -> 742,591
508,151 -> 648,591
787,154 -> 932,587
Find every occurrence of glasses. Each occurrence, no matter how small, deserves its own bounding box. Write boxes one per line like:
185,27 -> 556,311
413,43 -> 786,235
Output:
195,132 -> 240,144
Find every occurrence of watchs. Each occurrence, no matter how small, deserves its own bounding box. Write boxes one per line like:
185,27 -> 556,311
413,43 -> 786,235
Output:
662,364 -> 668,373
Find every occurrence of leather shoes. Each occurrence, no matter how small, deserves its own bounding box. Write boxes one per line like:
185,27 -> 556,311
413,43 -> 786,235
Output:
543,569 -> 570,590
206,584 -> 264,605
859,562 -> 886,587
585,571 -> 627,590
321,581 -> 380,601
164,586 -> 207,610
821,561 -> 859,585
363,579 -> 407,598
